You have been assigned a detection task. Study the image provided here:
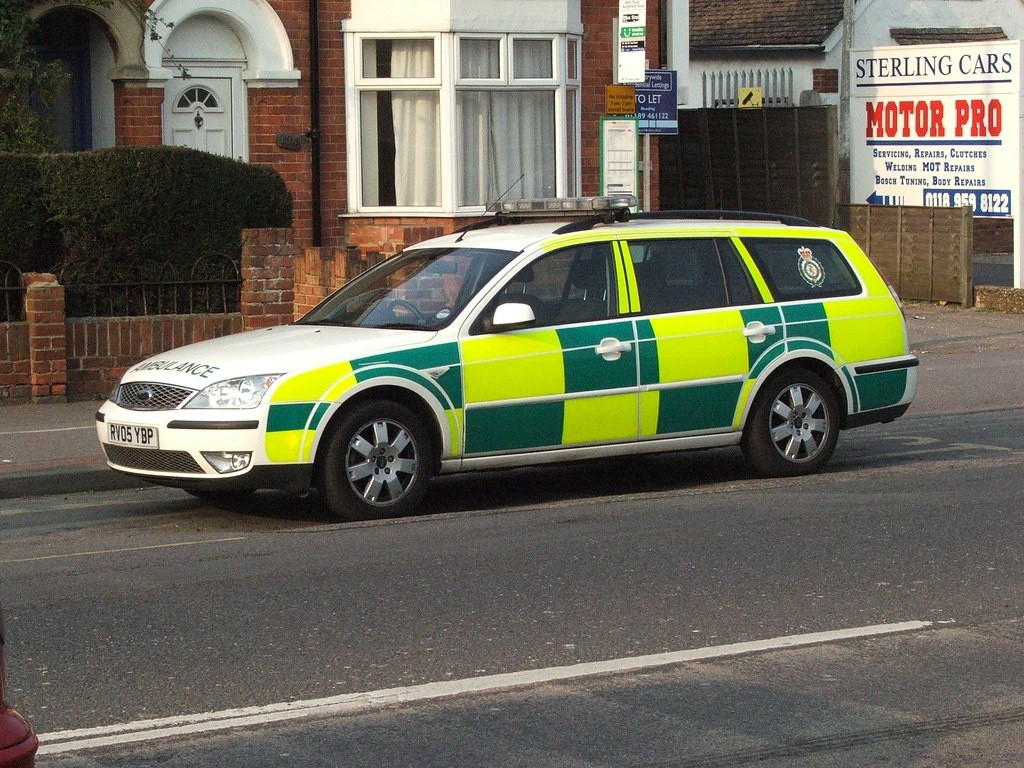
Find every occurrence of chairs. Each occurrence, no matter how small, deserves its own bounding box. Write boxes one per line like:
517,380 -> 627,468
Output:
493,263 -> 545,321
565,257 -> 608,323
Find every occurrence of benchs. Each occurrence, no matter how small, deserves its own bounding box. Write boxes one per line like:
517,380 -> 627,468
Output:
635,255 -> 742,310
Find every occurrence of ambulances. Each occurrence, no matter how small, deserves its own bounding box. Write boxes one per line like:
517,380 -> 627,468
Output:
93,208 -> 920,523
